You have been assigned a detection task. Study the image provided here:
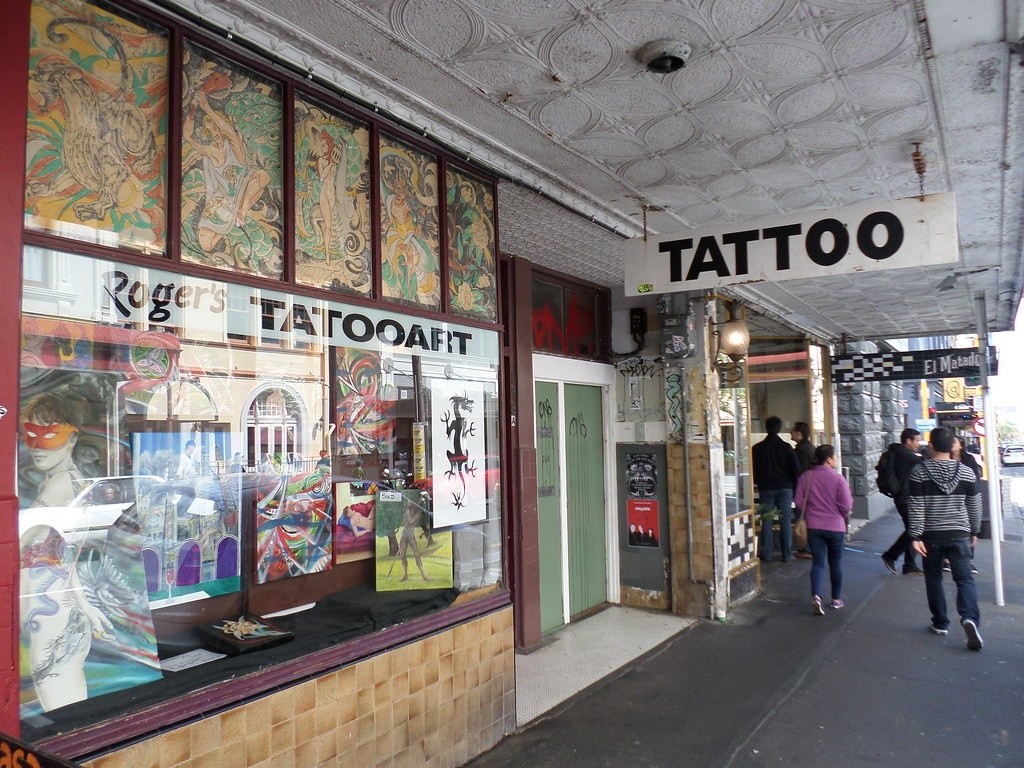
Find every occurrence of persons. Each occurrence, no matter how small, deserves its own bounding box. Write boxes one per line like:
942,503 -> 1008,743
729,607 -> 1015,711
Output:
752,416 -> 801,562
942,435 -> 979,574
99,485 -> 123,504
229,451 -> 247,473
875,428 -> 923,575
790,421 -> 816,558
351,458 -> 367,479
176,440 -> 196,478
907,427 -> 983,649
793,444 -> 854,616
316,450 -> 330,466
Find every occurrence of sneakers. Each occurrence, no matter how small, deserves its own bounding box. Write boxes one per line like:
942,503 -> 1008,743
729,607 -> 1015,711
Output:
929,622 -> 948,635
831,599 -> 844,609
812,595 -> 825,615
963,619 -> 983,650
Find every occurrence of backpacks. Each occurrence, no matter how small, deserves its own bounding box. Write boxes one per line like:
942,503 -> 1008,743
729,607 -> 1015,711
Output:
875,444 -> 913,498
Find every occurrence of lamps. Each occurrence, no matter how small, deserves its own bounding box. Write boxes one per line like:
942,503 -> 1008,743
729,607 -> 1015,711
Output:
834,382 -> 854,402
709,299 -> 750,383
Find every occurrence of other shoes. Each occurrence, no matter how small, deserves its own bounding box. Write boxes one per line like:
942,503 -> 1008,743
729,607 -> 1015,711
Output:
793,551 -> 812,558
784,555 -> 796,563
971,564 -> 978,574
881,552 -> 896,574
905,567 -> 924,575
942,562 -> 951,572
757,553 -> 764,561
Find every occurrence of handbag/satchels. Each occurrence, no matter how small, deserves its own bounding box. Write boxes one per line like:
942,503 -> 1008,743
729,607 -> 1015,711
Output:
793,470 -> 811,548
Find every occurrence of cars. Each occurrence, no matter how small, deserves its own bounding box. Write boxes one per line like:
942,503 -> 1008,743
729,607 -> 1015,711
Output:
90,471 -> 496,661
998,443 -> 1024,466
17,474 -> 182,569
408,455 -> 500,511
